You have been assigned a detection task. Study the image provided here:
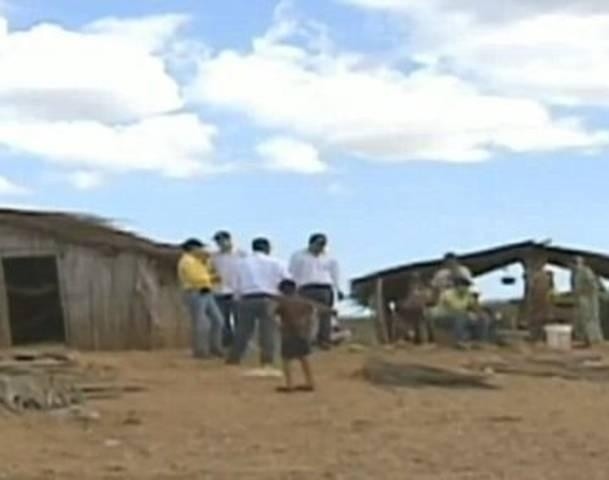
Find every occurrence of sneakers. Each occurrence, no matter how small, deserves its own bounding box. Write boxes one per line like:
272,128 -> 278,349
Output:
275,385 -> 314,393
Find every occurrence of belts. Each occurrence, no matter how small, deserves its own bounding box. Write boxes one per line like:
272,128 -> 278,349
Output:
223,294 -> 234,300
300,284 -> 331,291
243,293 -> 271,299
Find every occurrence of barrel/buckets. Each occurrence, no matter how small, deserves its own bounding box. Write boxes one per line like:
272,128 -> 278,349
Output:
543,324 -> 572,351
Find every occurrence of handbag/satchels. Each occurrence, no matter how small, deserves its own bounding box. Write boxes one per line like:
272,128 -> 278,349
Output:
199,287 -> 211,296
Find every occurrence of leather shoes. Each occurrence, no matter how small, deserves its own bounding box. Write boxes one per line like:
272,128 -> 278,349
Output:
192,337 -> 274,365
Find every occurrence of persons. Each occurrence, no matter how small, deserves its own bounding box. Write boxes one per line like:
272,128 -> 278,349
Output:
177,231 -> 344,365
401,253 -> 490,350
527,253 -> 602,349
273,280 -> 313,392
